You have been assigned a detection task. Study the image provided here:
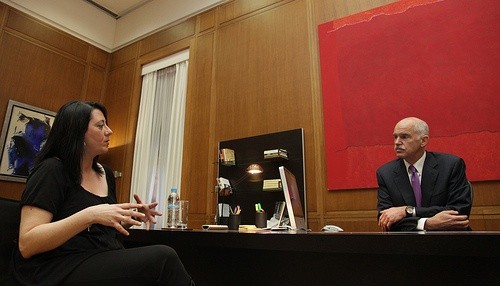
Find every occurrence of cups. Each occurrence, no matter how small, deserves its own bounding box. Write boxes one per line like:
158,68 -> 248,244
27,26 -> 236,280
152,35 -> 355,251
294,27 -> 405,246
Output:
228,214 -> 241,230
255,212 -> 267,228
178,201 -> 189,229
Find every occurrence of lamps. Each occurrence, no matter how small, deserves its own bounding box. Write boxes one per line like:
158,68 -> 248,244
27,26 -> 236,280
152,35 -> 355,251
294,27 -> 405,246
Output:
221,164 -> 264,225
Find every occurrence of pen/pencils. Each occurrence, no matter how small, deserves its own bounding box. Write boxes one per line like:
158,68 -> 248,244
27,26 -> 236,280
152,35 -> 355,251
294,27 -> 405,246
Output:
230,205 -> 241,214
255,203 -> 262,212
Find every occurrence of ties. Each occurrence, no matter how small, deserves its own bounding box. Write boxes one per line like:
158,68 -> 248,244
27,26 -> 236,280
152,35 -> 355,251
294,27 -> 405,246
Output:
409,165 -> 422,207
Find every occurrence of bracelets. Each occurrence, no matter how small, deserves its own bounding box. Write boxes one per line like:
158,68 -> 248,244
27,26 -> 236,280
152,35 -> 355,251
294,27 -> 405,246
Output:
132,210 -> 136,220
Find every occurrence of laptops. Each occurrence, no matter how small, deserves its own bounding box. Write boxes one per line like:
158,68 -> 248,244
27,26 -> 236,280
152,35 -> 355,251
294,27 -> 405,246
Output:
278,166 -> 306,232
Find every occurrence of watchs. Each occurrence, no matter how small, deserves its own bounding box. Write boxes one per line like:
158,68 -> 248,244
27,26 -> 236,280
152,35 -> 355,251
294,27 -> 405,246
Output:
406,206 -> 414,217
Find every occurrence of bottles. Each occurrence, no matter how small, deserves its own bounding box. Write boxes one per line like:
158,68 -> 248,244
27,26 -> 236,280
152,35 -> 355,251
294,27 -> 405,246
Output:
167,188 -> 179,228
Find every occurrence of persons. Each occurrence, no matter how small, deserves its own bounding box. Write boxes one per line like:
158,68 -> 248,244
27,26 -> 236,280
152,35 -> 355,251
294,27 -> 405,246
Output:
17,101 -> 193,286
376,116 -> 472,231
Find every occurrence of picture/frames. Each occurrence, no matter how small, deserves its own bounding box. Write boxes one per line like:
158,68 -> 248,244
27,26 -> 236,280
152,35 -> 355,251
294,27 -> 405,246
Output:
0,99 -> 57,183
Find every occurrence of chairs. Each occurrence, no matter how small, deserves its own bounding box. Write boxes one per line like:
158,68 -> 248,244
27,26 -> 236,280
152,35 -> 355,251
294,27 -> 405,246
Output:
0,196 -> 24,286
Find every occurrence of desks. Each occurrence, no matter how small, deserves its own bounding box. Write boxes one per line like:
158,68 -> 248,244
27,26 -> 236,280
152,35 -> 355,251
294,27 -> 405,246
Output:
128,226 -> 500,286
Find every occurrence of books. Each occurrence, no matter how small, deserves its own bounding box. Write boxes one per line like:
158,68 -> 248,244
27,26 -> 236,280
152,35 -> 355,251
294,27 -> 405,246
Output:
220,149 -> 235,165
263,179 -> 282,190
263,148 -> 288,158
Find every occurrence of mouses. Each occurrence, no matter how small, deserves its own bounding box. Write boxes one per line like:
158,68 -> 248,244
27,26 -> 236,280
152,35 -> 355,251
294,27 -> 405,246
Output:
322,224 -> 344,232
